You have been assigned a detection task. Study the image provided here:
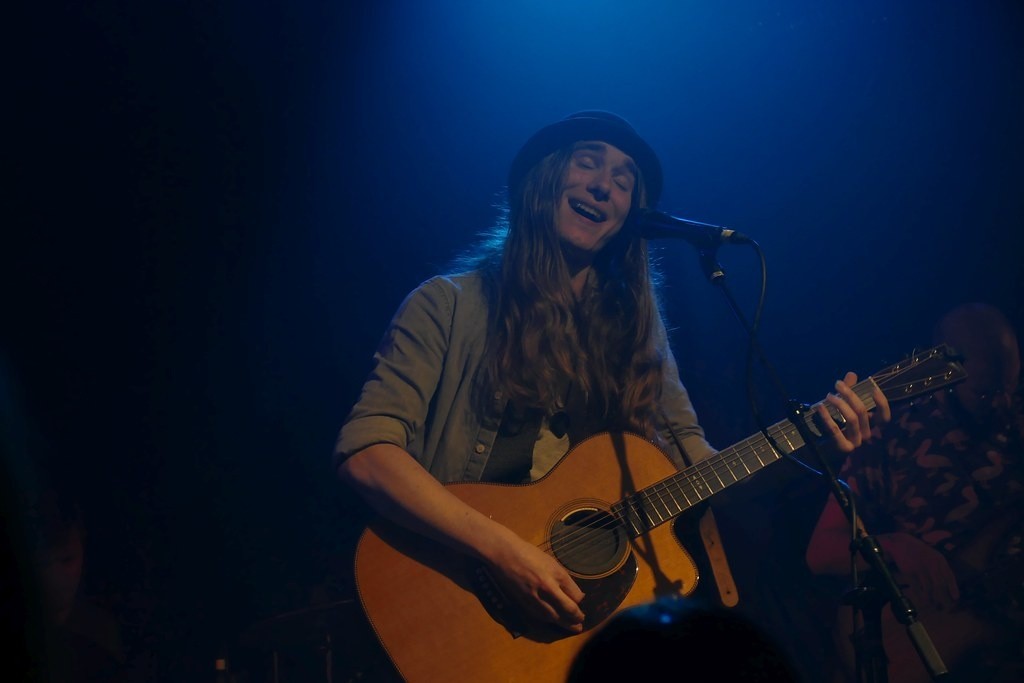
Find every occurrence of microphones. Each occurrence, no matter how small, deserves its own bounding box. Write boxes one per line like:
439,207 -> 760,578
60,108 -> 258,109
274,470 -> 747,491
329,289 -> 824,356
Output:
626,207 -> 750,245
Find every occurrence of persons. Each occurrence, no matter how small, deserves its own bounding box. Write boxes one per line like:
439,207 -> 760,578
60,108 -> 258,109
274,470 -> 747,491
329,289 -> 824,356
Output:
802,301 -> 1024,683
0,486 -> 124,683
335,110 -> 891,683
566,596 -> 802,683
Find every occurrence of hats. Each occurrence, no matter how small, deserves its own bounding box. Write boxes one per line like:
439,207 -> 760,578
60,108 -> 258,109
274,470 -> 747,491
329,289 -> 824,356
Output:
506,110 -> 663,209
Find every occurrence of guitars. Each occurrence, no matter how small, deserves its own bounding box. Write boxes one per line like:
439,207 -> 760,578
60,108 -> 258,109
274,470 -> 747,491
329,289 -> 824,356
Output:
350,343 -> 973,683
